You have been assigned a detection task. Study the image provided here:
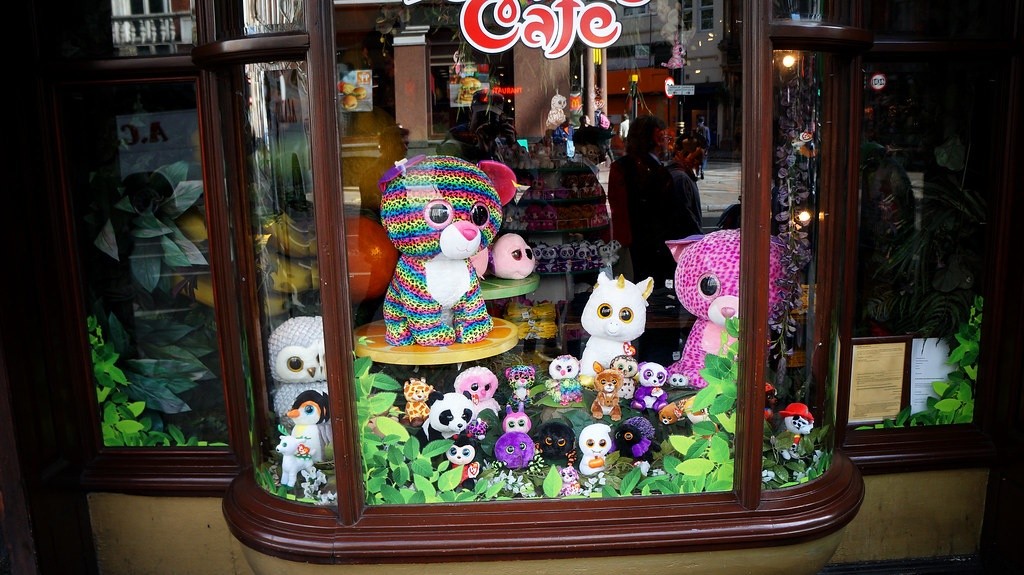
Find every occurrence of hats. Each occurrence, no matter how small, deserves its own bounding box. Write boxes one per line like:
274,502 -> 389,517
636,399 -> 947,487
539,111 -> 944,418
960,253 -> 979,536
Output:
572,126 -> 616,144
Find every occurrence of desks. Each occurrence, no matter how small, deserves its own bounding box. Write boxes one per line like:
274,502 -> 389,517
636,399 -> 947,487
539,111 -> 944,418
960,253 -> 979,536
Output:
474,272 -> 544,306
352,314 -> 517,390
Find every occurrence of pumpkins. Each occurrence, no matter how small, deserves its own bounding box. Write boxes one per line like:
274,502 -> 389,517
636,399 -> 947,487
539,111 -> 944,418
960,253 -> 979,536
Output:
342,210 -> 398,303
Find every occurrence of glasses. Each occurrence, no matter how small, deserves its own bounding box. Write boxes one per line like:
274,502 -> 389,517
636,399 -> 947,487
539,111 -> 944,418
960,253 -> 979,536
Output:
659,134 -> 673,140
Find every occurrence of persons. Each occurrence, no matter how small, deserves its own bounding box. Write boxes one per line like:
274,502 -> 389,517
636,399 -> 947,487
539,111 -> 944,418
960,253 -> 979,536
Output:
558,125 -> 613,279
618,113 -> 629,153
692,113 -> 712,183
439,88 -> 521,231
597,111 -> 616,164
352,126 -> 412,329
607,115 -> 671,252
667,131 -> 710,234
579,114 -> 592,128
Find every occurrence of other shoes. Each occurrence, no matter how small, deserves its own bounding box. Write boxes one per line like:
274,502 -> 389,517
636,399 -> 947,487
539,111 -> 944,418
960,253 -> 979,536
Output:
701,171 -> 704,179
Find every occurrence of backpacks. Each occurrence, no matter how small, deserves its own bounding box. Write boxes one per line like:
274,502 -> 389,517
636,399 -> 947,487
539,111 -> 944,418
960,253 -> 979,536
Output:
696,125 -> 707,140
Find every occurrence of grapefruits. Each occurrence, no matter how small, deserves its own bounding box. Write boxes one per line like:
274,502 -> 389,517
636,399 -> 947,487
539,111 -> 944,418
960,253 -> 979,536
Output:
345,105 -> 405,198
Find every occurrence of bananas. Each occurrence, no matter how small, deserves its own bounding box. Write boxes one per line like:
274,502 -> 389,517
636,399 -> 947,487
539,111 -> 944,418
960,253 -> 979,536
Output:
167,201 -> 319,316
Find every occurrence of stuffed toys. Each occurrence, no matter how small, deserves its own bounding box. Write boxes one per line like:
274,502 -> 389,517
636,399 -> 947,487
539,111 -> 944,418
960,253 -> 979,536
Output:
260,313 -> 820,496
494,141 -> 626,276
484,230 -> 532,281
379,152 -> 518,351
661,230 -> 809,391
578,273 -> 653,388
